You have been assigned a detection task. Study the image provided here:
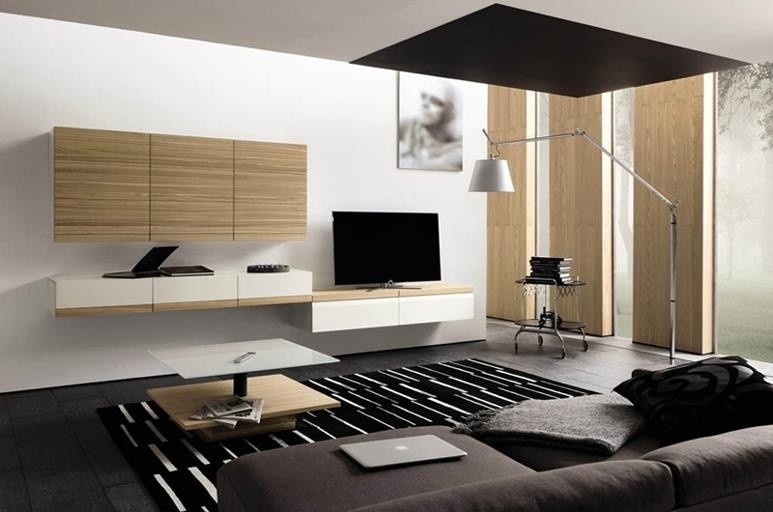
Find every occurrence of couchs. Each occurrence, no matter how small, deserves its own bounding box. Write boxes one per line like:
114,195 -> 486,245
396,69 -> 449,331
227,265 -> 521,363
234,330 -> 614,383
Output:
220,393 -> 772,511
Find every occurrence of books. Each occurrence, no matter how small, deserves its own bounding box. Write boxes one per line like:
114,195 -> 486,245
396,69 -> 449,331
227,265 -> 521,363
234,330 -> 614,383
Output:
529,257 -> 573,285
159,265 -> 215,277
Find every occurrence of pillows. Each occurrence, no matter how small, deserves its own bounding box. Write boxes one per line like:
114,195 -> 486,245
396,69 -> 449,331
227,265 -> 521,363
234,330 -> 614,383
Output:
612,355 -> 772,446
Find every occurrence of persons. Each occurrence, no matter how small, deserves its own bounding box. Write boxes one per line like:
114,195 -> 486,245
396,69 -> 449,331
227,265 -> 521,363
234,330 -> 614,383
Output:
397,77 -> 461,169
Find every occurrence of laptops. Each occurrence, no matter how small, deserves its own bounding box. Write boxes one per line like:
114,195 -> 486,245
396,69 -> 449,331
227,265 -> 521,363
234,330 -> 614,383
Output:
338,434 -> 467,469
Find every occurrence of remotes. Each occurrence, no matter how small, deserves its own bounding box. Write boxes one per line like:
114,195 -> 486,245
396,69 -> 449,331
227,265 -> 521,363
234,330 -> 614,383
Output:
234,351 -> 256,363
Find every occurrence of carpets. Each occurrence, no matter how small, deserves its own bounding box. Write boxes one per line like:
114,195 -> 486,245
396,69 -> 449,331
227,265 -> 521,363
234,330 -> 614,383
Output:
95,357 -> 605,511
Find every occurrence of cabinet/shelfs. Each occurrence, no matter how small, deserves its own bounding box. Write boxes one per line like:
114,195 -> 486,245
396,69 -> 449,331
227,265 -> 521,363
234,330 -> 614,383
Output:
513,278 -> 587,359
49,126 -> 307,244
49,267 -> 314,320
312,293 -> 475,333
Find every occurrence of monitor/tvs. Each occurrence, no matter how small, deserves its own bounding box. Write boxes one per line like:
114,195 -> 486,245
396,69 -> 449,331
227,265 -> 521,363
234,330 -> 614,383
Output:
333,211 -> 441,292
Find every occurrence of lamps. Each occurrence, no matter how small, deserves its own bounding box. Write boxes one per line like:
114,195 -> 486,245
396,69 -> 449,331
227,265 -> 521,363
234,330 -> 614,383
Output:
467,128 -> 679,366
